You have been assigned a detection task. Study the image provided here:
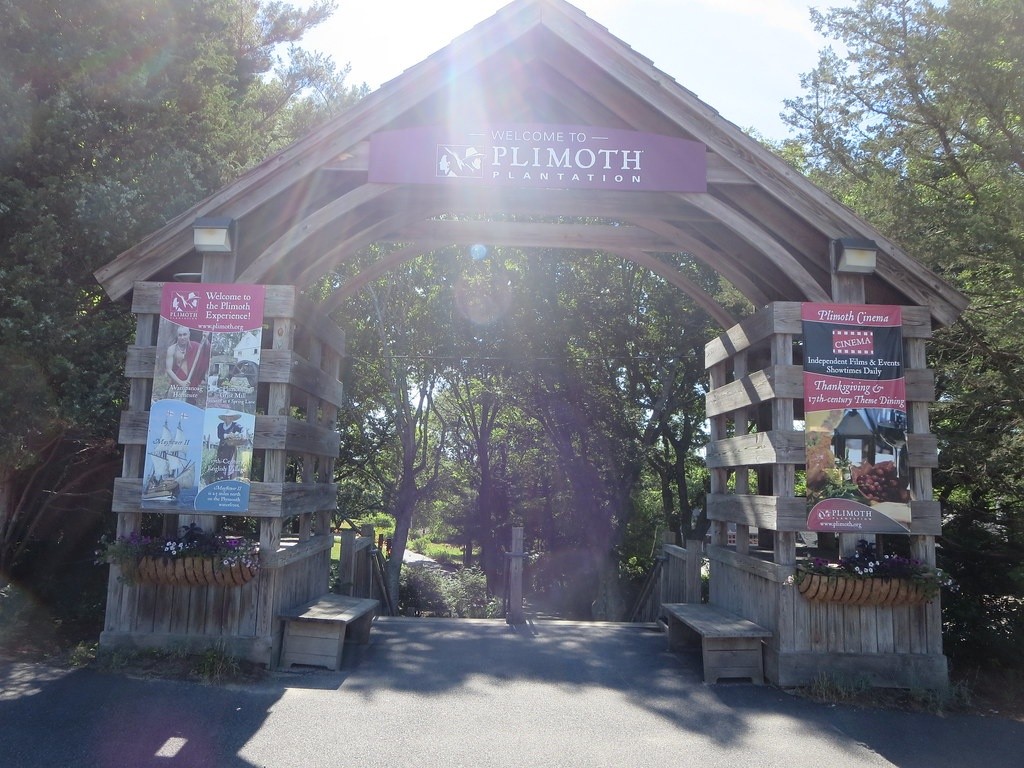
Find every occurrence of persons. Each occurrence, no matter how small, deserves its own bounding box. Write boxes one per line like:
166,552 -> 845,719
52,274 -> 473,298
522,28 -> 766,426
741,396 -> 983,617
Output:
215,409 -> 246,481
165,326 -> 211,406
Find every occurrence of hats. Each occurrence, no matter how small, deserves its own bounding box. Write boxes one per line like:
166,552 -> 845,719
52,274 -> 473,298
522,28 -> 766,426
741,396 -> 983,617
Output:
218,410 -> 241,421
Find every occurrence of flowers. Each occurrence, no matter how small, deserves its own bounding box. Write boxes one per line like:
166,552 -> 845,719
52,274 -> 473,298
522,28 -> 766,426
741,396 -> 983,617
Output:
93,523 -> 259,573
801,552 -> 961,594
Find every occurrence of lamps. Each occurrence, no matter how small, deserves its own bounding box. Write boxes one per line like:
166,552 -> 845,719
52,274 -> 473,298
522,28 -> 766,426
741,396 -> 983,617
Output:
834,237 -> 879,276
193,218 -> 235,254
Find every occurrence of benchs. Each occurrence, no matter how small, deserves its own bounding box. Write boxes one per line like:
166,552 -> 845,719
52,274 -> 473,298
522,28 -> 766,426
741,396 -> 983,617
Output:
661,603 -> 774,686
277,593 -> 380,673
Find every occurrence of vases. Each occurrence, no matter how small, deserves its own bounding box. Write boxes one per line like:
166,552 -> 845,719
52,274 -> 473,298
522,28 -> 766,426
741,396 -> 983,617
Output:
116,555 -> 257,585
796,564 -> 936,604
